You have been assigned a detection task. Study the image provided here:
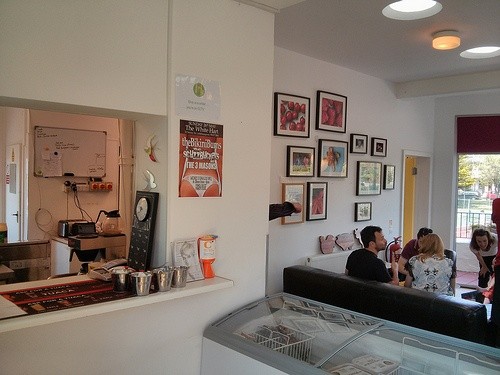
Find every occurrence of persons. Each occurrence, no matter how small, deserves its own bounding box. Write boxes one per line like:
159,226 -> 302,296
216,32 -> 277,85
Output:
470,228 -> 498,301
269,201 -> 303,221
398,228 -> 457,297
180,131 -> 222,197
346,225 -> 399,285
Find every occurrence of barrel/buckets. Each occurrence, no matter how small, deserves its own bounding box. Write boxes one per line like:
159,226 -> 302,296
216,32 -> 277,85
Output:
153,267 -> 175,291
109,266 -> 136,292
128,271 -> 157,296
170,266 -> 191,288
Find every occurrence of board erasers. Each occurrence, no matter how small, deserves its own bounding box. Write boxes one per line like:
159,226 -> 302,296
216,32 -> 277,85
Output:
64,173 -> 74,176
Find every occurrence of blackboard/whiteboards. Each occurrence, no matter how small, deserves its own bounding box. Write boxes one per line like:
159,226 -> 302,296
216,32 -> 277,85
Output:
34,125 -> 107,178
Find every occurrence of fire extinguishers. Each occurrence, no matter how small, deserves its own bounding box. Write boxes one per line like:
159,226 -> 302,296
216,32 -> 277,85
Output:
386,235 -> 403,273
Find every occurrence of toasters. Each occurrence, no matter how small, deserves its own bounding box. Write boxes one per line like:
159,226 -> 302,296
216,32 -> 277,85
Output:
58,218 -> 88,238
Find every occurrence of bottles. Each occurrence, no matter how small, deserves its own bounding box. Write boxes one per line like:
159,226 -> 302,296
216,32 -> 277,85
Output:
76,260 -> 96,276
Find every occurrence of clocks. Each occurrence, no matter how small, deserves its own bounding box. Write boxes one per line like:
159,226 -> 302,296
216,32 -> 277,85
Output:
135,198 -> 149,222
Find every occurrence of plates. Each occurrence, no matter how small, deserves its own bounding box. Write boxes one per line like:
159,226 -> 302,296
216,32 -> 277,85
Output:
77,233 -> 98,238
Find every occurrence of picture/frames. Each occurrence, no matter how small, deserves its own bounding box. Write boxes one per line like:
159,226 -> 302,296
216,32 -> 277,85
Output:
357,161 -> 382,196
282,183 -> 305,225
286,145 -> 315,177
314,90 -> 347,134
319,139 -> 350,179
372,137 -> 387,157
355,202 -> 371,222
349,133 -> 368,154
383,165 -> 396,190
306,181 -> 329,221
273,91 -> 311,139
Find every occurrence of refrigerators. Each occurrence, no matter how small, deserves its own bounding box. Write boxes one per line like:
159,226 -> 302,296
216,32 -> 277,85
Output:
200,290 -> 500,375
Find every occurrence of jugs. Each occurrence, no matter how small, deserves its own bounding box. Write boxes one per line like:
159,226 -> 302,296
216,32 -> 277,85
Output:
96,209 -> 122,235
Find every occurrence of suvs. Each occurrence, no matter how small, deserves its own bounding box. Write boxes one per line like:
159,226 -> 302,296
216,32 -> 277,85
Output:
458,191 -> 481,200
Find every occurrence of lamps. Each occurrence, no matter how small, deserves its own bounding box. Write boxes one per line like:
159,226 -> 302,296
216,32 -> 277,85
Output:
432,31 -> 461,50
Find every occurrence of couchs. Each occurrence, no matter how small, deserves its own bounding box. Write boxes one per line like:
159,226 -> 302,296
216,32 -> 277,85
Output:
283,265 -> 500,352
